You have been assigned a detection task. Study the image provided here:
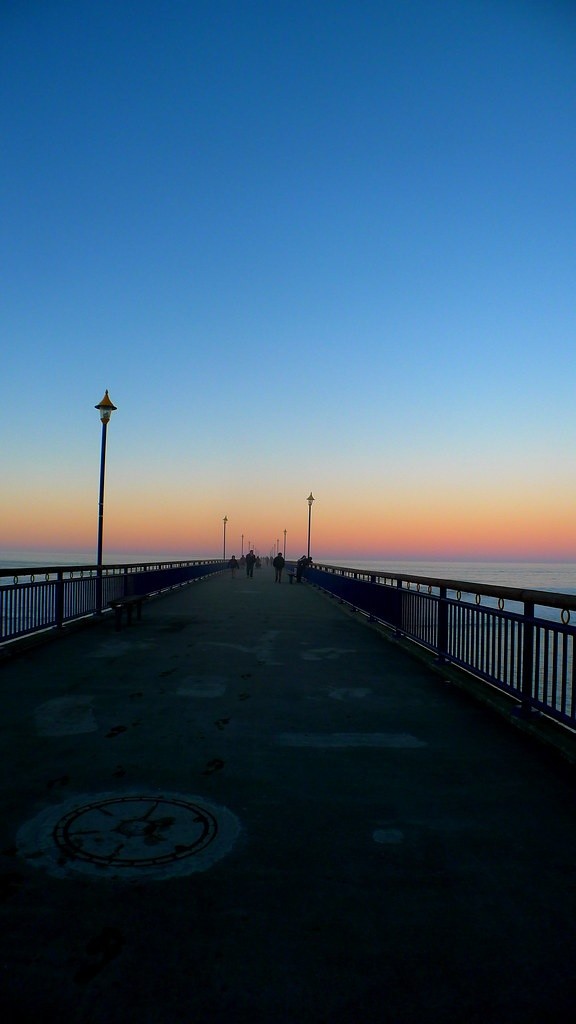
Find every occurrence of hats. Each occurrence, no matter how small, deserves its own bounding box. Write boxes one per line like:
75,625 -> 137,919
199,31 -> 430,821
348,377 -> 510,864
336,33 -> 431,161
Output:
278,552 -> 282,555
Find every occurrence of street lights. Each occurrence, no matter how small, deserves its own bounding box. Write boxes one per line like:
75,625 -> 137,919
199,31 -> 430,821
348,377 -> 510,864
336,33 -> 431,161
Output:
241,534 -> 244,554
248,541 -> 251,550
93,389 -> 118,617
283,529 -> 287,561
307,491 -> 315,560
277,539 -> 279,555
223,515 -> 228,561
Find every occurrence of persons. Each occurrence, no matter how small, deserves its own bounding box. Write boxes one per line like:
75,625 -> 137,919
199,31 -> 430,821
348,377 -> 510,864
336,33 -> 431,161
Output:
255,556 -> 261,568
246,550 -> 256,579
272,553 -> 285,584
260,556 -> 264,561
228,556 -> 239,579
266,556 -> 269,566
294,555 -> 313,585
240,555 -> 246,570
270,556 -> 274,566
